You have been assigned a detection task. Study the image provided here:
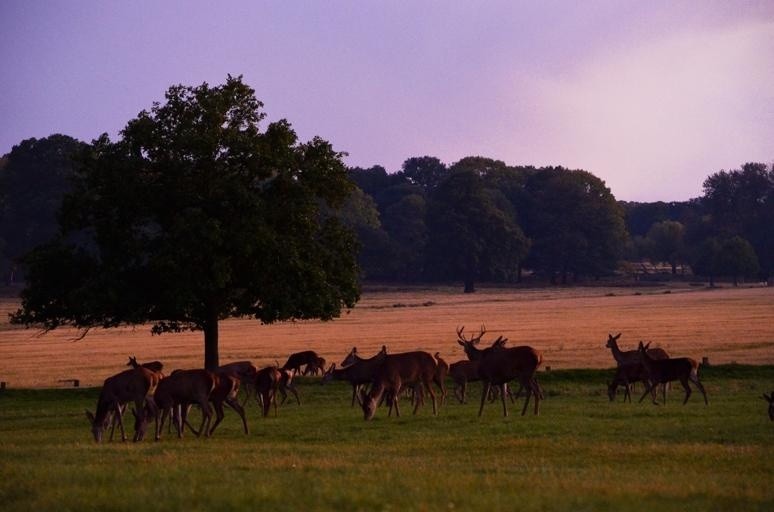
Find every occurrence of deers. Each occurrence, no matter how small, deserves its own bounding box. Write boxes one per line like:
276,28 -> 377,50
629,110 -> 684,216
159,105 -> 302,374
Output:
320,320 -> 544,423
82,350 -> 327,443
607,333 -> 709,406
